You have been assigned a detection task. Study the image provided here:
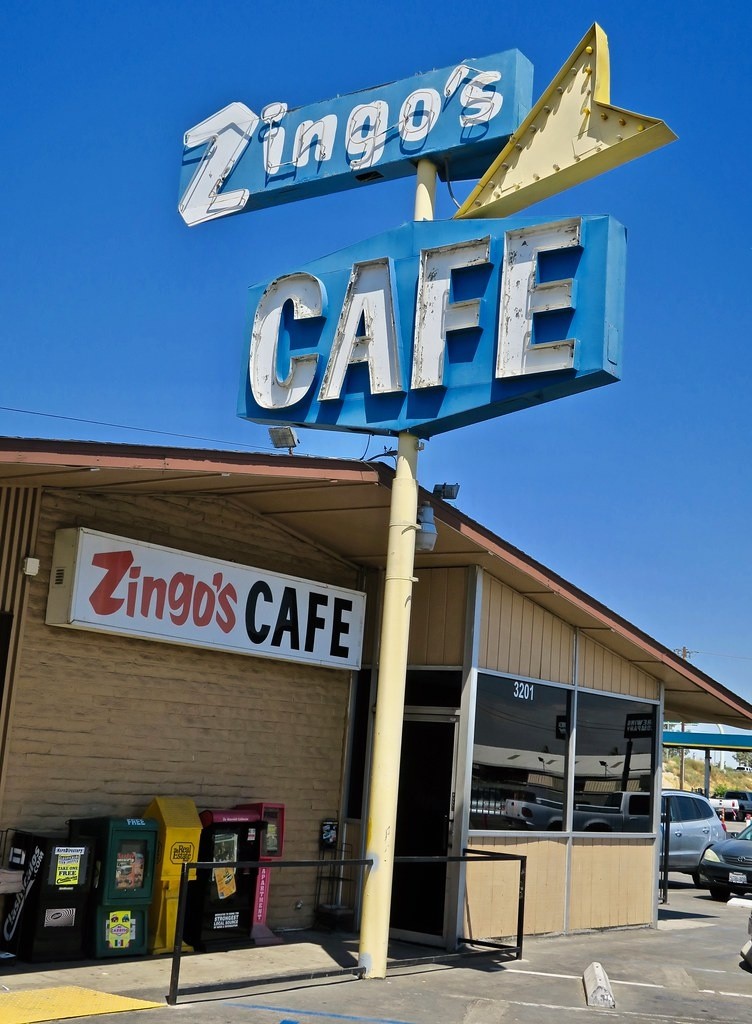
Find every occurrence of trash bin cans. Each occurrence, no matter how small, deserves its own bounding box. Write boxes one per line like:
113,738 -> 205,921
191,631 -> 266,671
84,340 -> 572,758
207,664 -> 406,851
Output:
0,794 -> 284,964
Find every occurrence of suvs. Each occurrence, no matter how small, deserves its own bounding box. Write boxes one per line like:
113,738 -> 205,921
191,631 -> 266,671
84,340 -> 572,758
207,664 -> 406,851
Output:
662,790 -> 728,889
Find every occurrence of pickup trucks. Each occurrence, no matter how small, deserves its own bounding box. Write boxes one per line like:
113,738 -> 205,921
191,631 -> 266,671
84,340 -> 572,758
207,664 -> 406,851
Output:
735,766 -> 751,773
708,792 -> 752,819
504,790 -> 652,834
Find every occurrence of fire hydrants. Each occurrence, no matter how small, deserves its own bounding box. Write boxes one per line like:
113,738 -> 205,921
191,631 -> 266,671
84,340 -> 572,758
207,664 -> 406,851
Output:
744,814 -> 752,827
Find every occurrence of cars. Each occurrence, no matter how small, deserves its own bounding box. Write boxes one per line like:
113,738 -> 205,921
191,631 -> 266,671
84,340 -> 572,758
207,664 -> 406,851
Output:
699,820 -> 752,899
